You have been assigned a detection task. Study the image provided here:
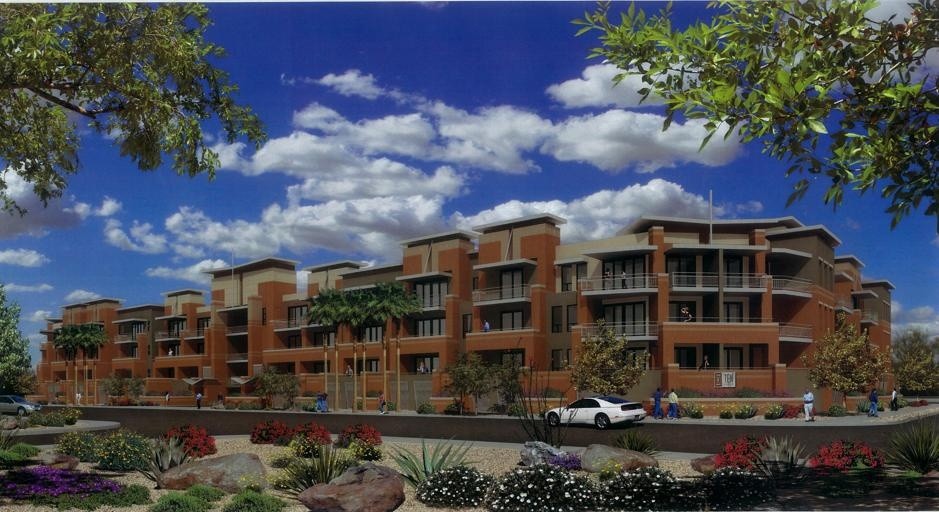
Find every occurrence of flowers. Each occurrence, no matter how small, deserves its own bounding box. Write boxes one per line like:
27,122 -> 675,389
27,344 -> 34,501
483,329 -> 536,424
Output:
58,407 -> 83,422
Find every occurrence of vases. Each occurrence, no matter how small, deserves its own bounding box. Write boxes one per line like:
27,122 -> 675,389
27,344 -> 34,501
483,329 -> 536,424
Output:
66,419 -> 76,425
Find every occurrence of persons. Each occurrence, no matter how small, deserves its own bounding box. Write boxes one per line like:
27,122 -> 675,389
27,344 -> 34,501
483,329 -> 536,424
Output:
866,387 -> 879,417
702,355 -> 711,368
195,391 -> 202,409
165,390 -> 170,407
891,387 -> 898,411
653,387 -> 664,419
666,388 -> 680,420
168,347 -> 173,357
345,365 -> 354,378
377,391 -> 387,415
620,267 -> 628,289
322,392 -> 328,413
417,362 -> 429,374
604,267 -> 612,289
803,388 -> 815,421
681,307 -> 692,322
483,319 -> 489,332
316,390 -> 322,411
76,392 -> 82,406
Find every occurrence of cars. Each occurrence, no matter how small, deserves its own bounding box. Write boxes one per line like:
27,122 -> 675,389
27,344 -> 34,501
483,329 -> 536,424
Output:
0,395 -> 42,416
544,396 -> 647,429
